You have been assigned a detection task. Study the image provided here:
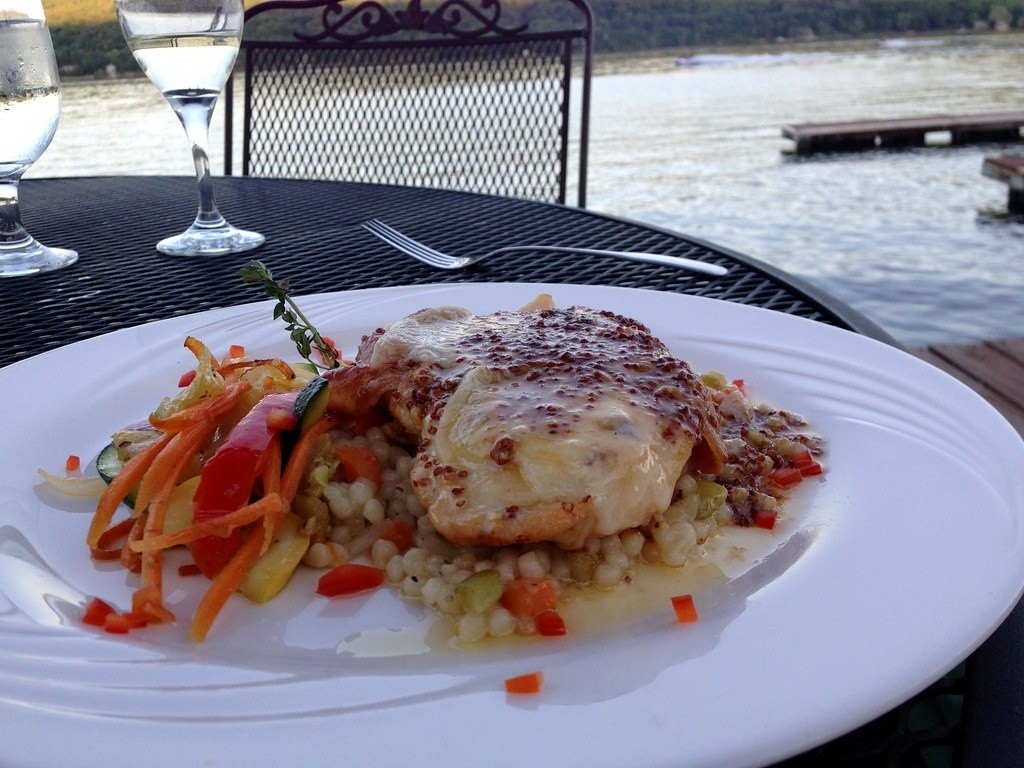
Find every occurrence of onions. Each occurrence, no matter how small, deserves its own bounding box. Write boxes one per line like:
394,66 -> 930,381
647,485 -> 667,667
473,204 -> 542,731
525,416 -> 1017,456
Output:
36,468 -> 109,497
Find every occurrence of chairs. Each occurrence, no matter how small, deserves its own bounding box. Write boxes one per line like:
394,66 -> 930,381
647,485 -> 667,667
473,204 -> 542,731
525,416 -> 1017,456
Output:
224,0 -> 595,210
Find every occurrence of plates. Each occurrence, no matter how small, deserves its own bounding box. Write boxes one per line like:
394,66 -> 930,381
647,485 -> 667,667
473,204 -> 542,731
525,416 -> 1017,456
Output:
1,278 -> 1024,768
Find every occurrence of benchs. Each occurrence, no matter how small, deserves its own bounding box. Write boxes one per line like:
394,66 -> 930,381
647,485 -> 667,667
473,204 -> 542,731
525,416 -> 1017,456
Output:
977,154 -> 1024,224
780,111 -> 1024,161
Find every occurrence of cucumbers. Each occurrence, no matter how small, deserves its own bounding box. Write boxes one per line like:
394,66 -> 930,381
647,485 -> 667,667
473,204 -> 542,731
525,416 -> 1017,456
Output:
96,442 -> 136,510
279,376 -> 329,479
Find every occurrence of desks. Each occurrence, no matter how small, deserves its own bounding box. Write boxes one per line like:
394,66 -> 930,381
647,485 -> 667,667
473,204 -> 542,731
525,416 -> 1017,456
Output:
0,175 -> 1024,768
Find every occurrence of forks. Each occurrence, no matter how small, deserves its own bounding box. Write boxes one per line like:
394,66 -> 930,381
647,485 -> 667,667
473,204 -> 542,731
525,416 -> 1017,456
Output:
359,218 -> 728,280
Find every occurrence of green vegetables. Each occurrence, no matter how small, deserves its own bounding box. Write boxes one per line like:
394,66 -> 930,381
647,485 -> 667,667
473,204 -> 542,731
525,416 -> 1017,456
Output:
239,260 -> 340,372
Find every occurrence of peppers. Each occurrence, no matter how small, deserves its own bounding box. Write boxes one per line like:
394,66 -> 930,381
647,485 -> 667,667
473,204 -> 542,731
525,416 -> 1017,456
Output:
84,336 -> 823,693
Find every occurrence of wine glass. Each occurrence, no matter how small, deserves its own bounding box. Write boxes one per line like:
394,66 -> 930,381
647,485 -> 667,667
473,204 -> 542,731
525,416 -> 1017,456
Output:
112,0 -> 265,258
0,0 -> 80,281
